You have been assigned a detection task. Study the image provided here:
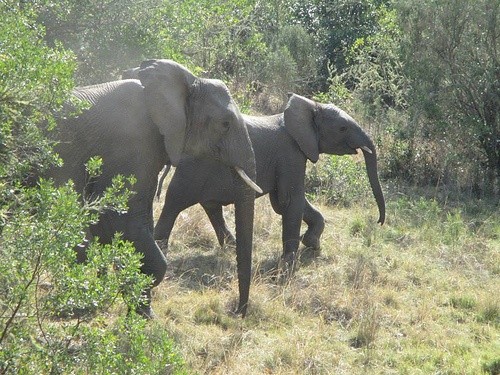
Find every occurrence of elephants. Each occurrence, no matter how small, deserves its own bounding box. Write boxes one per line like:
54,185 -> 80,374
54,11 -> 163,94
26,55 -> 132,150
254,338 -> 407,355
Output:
0,58 -> 264,320
152,94 -> 386,285
